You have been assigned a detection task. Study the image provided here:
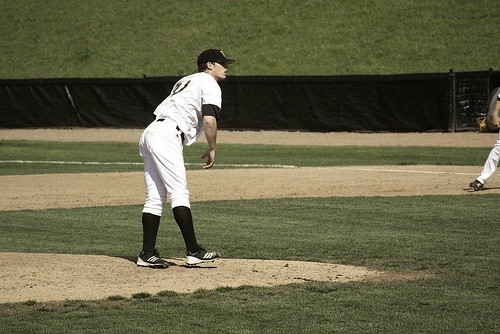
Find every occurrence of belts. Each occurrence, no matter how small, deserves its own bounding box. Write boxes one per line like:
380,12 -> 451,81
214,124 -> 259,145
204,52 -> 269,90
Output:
157,118 -> 185,145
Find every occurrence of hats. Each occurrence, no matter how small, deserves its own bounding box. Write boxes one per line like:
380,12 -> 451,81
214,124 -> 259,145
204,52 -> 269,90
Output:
197,48 -> 235,65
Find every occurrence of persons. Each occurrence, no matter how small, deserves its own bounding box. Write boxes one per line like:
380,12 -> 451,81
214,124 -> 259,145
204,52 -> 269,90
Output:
469,88 -> 500,191
137,48 -> 237,267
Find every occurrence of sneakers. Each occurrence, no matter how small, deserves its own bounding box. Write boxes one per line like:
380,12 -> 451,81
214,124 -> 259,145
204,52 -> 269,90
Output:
469,181 -> 482,191
186,246 -> 221,267
136,251 -> 170,268
